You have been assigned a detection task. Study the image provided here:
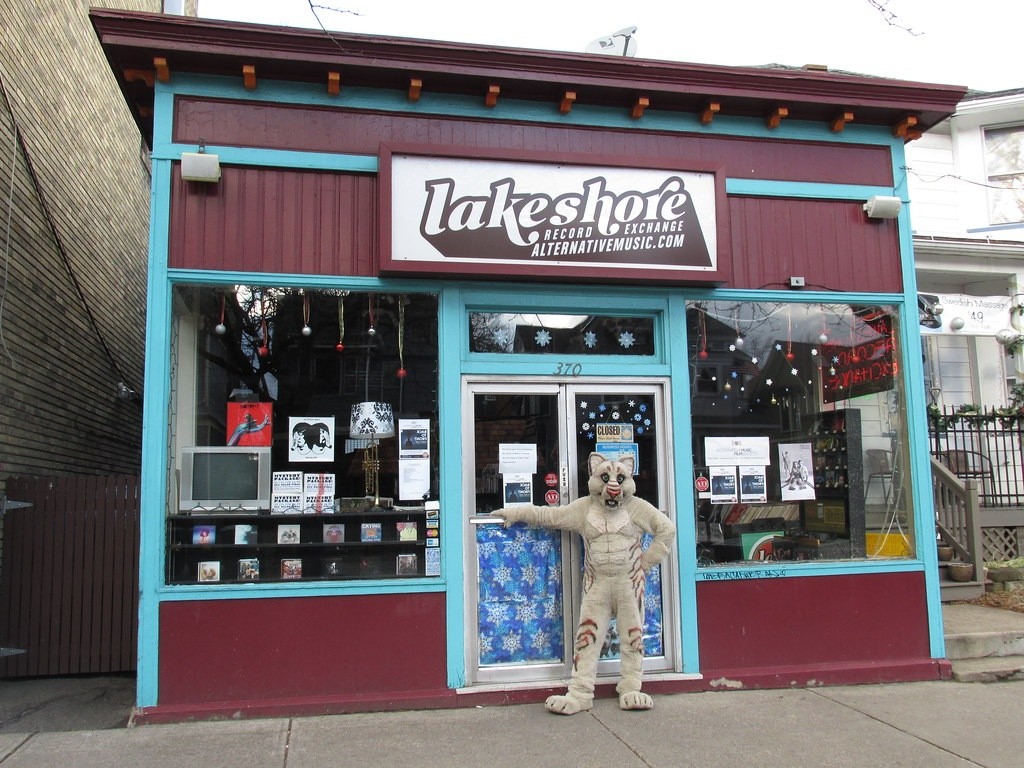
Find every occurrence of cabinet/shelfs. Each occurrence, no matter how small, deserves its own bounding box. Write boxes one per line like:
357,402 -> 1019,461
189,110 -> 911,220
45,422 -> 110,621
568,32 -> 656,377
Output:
163,510 -> 439,585
791,409 -> 868,558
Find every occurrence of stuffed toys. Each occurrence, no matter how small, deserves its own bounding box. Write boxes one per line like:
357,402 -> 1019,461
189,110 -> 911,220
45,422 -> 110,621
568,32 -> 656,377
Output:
497,451 -> 675,715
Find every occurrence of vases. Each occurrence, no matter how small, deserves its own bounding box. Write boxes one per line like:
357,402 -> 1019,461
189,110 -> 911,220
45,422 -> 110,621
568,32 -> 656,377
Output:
947,563 -> 973,582
938,547 -> 953,561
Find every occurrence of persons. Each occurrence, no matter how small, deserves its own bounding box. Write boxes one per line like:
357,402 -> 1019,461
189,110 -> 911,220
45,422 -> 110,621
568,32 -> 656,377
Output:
782,451 -> 809,491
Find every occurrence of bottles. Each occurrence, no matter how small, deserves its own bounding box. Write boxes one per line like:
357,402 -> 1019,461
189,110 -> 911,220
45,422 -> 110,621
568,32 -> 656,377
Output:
816,502 -> 824,522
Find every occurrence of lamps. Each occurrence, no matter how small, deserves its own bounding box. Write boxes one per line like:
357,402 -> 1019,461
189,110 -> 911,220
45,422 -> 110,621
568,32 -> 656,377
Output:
180,152 -> 221,183
349,402 -> 396,510
862,195 -> 902,218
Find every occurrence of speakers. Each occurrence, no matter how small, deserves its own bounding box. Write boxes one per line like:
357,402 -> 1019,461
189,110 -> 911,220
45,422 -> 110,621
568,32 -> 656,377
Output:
180,152 -> 220,182
866,196 -> 901,218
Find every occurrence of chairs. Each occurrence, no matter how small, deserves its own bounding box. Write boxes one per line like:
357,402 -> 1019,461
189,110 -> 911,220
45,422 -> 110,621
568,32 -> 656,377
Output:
932,449 -> 999,507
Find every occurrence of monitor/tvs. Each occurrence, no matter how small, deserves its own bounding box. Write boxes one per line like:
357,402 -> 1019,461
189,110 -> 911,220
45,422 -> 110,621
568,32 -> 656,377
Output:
178,446 -> 270,514
